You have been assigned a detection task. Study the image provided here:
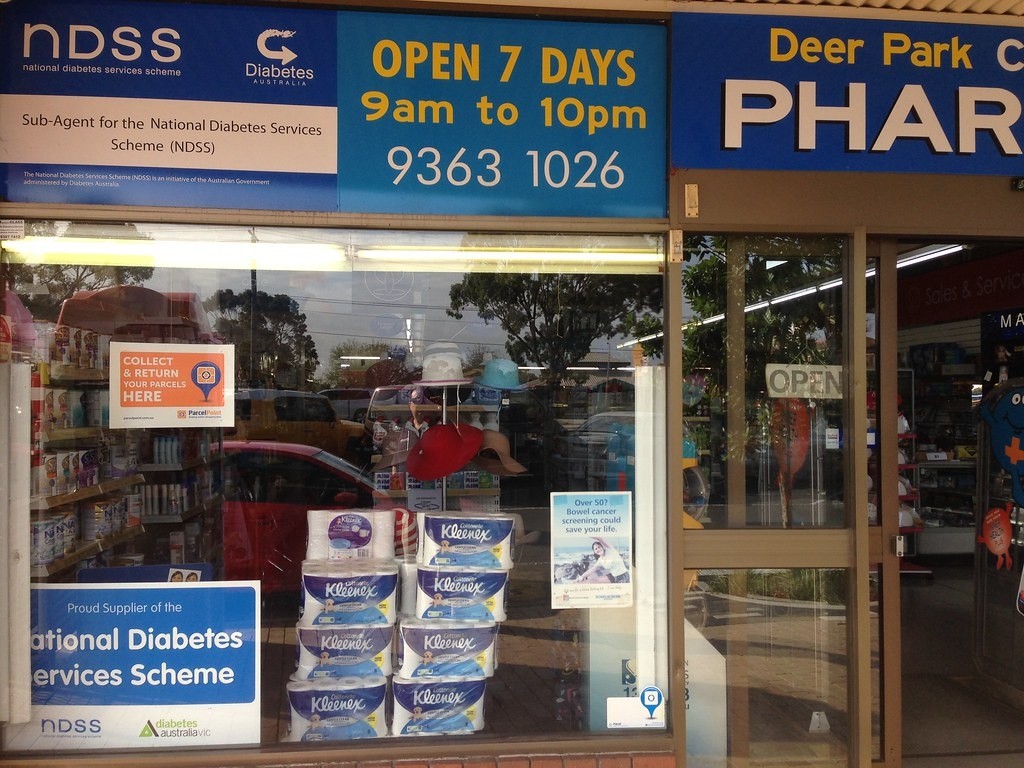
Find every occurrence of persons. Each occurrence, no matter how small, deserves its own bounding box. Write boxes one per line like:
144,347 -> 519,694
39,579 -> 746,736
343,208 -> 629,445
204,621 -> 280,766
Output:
572,536 -> 630,584
321,597 -> 338,614
438,539 -> 453,556
408,704 -> 427,723
316,651 -> 333,669
422,650 -> 438,666
430,592 -> 444,608
306,713 -> 324,734
170,571 -> 199,582
398,387 -> 444,440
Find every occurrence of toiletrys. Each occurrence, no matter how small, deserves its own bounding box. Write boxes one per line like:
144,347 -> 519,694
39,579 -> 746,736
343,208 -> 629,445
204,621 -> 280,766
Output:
153,435 -> 181,465
194,468 -> 218,504
132,484 -> 189,515
197,427 -> 220,456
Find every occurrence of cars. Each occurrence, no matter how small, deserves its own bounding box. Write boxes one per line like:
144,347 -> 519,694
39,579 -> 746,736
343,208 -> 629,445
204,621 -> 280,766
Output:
498,419 -> 627,507
209,440 -> 395,603
551,401 -> 703,496
316,386 -> 372,427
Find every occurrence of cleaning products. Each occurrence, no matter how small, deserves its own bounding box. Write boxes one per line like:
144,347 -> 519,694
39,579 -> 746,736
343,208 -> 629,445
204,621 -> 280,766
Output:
373,412 -> 499,455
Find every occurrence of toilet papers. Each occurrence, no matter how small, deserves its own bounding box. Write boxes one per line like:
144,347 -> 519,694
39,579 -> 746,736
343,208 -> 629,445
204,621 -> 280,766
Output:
288,511 -> 516,738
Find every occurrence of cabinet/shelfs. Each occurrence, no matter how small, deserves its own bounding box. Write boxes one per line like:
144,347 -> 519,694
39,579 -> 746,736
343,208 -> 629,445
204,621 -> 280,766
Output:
371,387 -> 503,512
28,324 -> 247,581
834,343 -> 1024,577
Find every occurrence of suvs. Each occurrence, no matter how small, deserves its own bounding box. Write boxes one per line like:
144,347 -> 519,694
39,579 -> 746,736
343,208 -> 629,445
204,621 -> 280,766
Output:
219,387 -> 373,470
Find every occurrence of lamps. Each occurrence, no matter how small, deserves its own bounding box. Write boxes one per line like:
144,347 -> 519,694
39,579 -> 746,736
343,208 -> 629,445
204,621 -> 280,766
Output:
0,222 -> 351,274
353,230 -> 669,275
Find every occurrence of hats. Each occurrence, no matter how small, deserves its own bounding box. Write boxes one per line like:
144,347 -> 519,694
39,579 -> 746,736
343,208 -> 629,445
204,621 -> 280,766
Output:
472,429 -> 528,476
472,359 -> 524,391
411,342 -> 474,387
365,429 -> 419,476
406,423 -> 484,482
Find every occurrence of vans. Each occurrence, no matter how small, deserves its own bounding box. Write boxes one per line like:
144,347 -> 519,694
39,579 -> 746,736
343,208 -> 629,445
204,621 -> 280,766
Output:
364,380 -> 479,439
